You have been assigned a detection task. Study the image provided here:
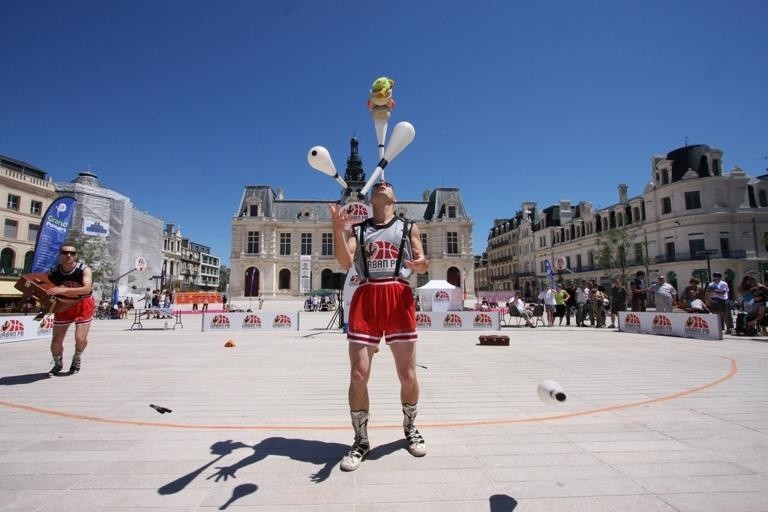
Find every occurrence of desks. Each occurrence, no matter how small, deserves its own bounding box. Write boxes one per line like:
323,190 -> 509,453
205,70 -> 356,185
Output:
308,303 -> 335,310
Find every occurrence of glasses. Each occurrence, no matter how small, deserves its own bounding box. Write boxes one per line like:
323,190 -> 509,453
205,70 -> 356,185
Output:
372,183 -> 393,189
59,250 -> 76,256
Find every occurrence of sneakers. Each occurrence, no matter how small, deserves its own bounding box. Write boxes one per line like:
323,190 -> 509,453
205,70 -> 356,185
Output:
403,425 -> 428,457
722,326 -> 768,337
340,438 -> 370,473
525,323 -> 616,329
48,363 -> 63,376
69,354 -> 82,376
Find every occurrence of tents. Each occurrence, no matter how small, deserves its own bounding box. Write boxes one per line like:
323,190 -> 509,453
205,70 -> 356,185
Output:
415,280 -> 463,311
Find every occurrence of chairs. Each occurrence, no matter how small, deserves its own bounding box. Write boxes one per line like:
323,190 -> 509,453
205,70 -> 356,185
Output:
500,299 -> 547,331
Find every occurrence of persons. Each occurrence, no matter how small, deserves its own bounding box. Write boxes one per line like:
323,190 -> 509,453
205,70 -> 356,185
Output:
304,293 -> 335,312
99,296 -> 133,319
222,295 -> 227,309
201,297 -> 209,311
192,297 -> 198,312
538,271 -> 768,336
509,292 -> 536,328
481,303 -> 488,310
47,244 -> 95,377
138,287 -> 174,319
482,297 -> 488,305
328,182 -> 428,472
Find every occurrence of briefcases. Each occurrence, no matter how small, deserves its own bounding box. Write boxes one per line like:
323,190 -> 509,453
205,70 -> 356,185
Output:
479,335 -> 510,346
13,271 -> 79,314
